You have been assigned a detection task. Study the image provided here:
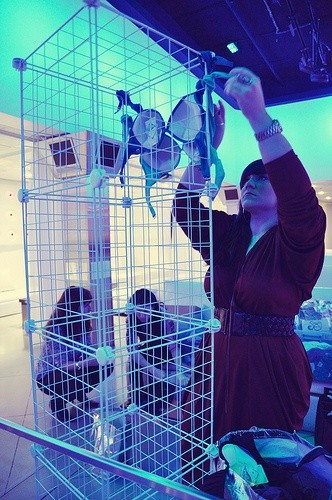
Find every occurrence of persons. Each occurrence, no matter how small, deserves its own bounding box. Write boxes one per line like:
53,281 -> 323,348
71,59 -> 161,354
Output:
120,289 -> 189,413
171,68 -> 326,490
36,286 -> 114,422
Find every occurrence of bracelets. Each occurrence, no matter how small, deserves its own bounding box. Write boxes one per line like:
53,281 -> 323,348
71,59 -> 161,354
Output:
75,363 -> 82,371
254,119 -> 283,142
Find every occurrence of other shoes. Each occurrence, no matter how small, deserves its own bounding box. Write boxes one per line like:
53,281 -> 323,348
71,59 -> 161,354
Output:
76,391 -> 100,407
51,396 -> 69,420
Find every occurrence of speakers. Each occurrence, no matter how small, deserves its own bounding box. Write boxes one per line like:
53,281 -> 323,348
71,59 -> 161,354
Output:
315,386 -> 332,463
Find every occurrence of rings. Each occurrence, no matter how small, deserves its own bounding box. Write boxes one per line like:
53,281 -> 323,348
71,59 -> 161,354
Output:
216,122 -> 225,126
235,73 -> 254,85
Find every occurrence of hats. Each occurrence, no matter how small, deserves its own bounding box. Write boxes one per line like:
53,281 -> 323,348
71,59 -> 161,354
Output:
293,446 -> 332,500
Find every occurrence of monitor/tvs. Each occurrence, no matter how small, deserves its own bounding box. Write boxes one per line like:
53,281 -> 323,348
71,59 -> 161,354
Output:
95,140 -> 121,169
224,188 -> 240,201
48,137 -> 79,169
